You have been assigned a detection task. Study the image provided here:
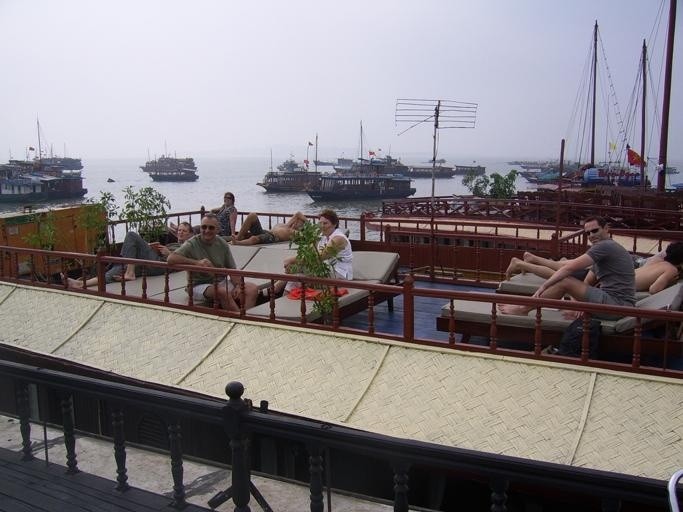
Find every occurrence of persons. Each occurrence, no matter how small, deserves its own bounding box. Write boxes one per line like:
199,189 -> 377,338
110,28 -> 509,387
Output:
231,211 -> 310,245
256,208 -> 354,300
504,241 -> 683,294
165,214 -> 259,312
59,222 -> 193,289
170,192 -> 236,240
497,215 -> 636,321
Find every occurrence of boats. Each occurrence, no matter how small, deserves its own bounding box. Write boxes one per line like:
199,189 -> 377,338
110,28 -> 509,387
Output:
0,157 -> 87,203
140,155 -> 199,181
256,158 -> 486,203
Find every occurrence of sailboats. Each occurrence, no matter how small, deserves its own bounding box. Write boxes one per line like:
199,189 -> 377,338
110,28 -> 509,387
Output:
516,19 -> 662,187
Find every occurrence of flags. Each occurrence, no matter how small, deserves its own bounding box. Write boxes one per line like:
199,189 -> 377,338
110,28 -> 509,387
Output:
368,151 -> 374,156
627,149 -> 646,167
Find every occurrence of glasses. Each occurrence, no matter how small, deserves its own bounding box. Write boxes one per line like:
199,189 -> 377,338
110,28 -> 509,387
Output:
201,224 -> 217,231
585,228 -> 600,235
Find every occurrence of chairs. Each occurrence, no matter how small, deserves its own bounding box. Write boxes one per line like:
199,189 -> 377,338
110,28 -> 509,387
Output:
436,271 -> 683,365
